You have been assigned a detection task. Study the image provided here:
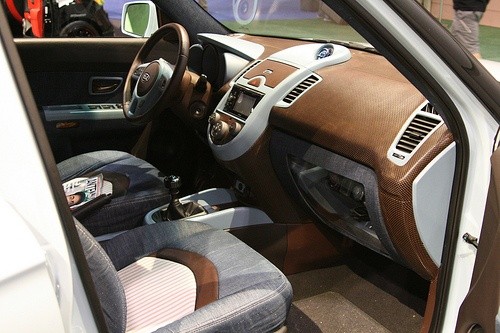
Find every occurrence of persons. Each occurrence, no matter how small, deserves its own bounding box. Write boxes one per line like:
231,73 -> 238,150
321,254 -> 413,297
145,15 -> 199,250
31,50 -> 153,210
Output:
449,1 -> 489,62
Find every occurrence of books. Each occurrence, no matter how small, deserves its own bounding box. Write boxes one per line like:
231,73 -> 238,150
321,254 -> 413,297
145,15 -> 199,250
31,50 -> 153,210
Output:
61,174 -> 113,216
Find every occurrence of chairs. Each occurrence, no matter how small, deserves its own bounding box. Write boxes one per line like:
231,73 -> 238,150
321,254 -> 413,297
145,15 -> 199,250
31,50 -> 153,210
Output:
69,214 -> 293,332
55,149 -> 171,235
